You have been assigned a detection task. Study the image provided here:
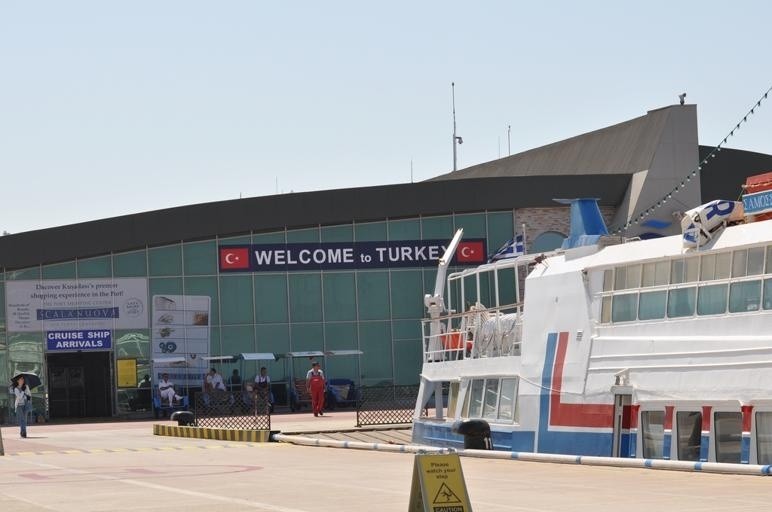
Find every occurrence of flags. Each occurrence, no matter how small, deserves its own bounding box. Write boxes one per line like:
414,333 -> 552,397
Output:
487,233 -> 523,263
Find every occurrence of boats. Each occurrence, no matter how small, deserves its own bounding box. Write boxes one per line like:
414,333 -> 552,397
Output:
413,198 -> 772,474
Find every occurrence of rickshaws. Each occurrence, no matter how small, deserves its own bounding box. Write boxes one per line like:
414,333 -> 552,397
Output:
239,352 -> 275,413
201,356 -> 235,417
326,350 -> 363,411
285,351 -> 325,412
151,357 -> 189,419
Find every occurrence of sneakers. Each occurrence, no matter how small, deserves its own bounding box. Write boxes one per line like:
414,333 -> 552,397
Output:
170,404 -> 174,409
314,413 -> 318,416
176,397 -> 184,401
319,411 -> 322,415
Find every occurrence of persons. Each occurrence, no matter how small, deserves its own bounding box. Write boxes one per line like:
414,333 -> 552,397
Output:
139,375 -> 151,387
14,376 -> 32,438
305,361 -> 327,418
228,369 -> 244,391
252,367 -> 275,415
159,372 -> 185,408
206,367 -> 226,391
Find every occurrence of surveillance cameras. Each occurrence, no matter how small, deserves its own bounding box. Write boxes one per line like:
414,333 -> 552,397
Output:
458,139 -> 463,144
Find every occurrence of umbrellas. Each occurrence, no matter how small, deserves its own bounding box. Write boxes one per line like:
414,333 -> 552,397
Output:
11,372 -> 41,391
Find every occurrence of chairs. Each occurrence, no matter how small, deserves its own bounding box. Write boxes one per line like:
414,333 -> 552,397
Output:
153,379 -> 361,419
